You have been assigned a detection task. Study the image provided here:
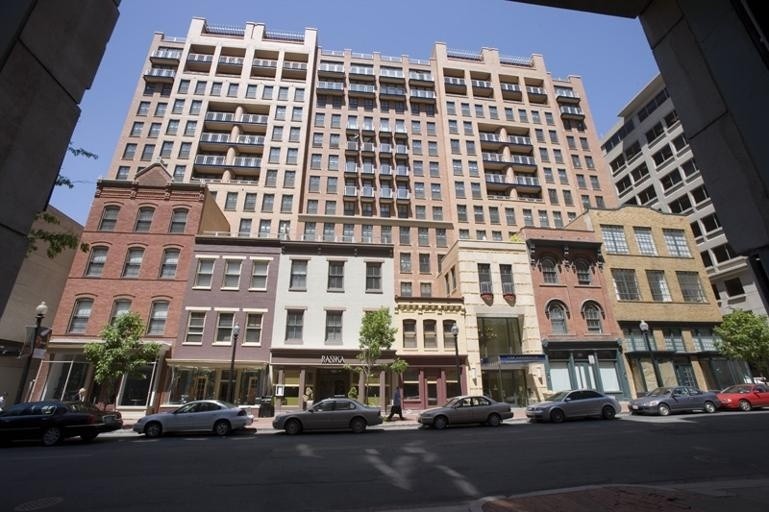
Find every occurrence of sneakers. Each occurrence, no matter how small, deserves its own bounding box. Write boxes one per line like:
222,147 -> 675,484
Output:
400,416 -> 403,420
388,417 -> 391,420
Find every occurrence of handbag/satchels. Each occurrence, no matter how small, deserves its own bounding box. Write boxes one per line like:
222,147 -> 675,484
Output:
391,406 -> 401,414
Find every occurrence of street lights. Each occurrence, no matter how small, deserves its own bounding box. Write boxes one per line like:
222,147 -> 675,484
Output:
14,301 -> 49,405
451,323 -> 461,396
638,319 -> 665,387
226,321 -> 240,402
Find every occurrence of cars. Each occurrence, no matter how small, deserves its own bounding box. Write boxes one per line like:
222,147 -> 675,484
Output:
418,394 -> 513,430
272,397 -> 384,435
627,385 -> 721,417
1,398 -> 124,447
132,399 -> 255,439
525,388 -> 621,424
716,383 -> 768,412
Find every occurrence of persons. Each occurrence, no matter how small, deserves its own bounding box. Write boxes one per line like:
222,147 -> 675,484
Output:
0,391 -> 9,411
72,387 -> 88,410
387,386 -> 405,420
